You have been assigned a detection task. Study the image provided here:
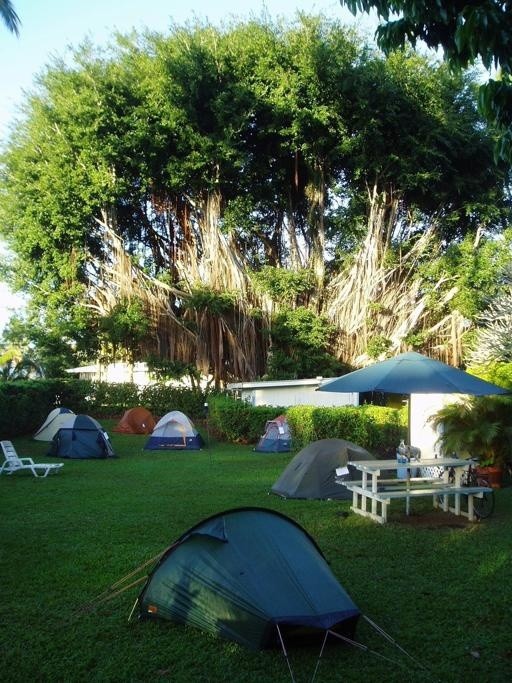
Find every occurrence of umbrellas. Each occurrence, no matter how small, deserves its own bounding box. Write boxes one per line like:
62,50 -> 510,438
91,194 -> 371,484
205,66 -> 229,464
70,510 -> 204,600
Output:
314,348 -> 512,517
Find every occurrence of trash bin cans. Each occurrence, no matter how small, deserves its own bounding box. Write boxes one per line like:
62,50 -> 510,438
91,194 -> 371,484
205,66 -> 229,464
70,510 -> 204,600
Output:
396,455 -> 417,479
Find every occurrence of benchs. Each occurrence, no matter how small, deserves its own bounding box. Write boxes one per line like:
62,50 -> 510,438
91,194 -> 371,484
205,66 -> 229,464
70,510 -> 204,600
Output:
334,475 -> 494,527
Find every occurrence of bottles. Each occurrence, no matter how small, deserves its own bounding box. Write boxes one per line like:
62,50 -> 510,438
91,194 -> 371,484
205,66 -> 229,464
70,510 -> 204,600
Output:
396,439 -> 408,479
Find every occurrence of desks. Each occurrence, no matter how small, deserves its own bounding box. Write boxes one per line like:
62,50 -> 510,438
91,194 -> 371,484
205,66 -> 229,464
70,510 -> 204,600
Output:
347,457 -> 474,518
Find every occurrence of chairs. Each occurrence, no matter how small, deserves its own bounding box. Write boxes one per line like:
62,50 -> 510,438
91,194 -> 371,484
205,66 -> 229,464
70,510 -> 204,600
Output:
1,439 -> 65,477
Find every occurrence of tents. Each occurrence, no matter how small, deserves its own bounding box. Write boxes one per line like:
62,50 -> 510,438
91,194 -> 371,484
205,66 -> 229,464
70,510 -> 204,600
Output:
253,413 -> 293,453
33,408 -> 76,441
145,410 -> 205,452
113,407 -> 154,433
129,505 -> 428,683
48,415 -> 114,459
272,436 -> 379,500
